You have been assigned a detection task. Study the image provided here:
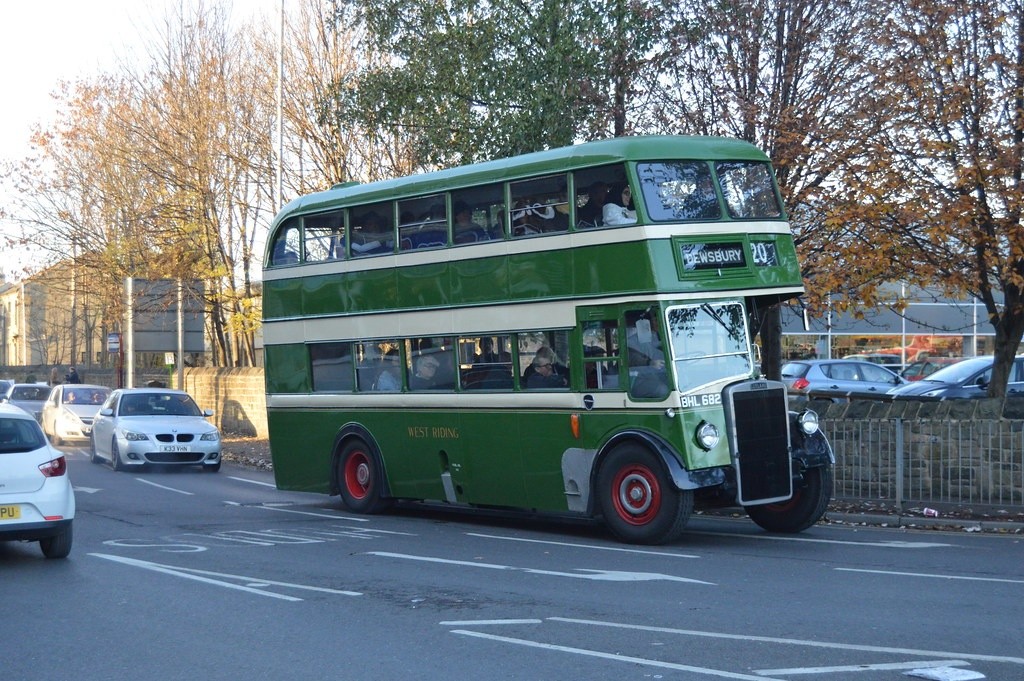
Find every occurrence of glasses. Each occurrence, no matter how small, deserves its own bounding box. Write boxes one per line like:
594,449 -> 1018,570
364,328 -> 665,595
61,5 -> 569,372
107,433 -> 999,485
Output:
622,190 -> 631,197
422,364 -> 437,369
537,363 -> 553,370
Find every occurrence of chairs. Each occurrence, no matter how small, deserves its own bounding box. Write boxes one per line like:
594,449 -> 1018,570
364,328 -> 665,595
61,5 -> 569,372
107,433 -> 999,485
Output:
137,403 -> 154,413
843,370 -> 854,380
345,216 -> 601,256
832,369 -> 838,379
0,428 -> 18,444
164,400 -> 178,412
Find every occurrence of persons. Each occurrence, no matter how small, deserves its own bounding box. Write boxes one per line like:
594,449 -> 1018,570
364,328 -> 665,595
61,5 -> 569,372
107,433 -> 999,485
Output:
68,392 -> 77,401
680,171 -> 740,221
65,367 -> 79,384
26,370 -> 37,384
629,309 -> 689,396
362,313 -> 609,390
351,181 -> 644,256
93,392 -> 102,402
49,367 -> 61,387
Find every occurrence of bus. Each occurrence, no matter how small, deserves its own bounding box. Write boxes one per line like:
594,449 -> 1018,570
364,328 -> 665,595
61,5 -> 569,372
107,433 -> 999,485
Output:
259,132 -> 835,547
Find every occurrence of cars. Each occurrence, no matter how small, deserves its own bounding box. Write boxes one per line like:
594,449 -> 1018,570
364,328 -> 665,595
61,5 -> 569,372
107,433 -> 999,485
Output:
778,350 -> 1024,401
88,385 -> 222,474
0,383 -> 53,421
0,401 -> 77,560
0,380 -> 12,402
39,383 -> 115,447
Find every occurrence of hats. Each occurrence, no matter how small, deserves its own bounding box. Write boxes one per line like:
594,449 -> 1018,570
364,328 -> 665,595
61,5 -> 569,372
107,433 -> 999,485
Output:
582,346 -> 605,356
